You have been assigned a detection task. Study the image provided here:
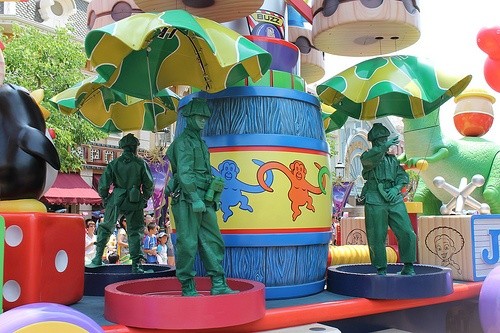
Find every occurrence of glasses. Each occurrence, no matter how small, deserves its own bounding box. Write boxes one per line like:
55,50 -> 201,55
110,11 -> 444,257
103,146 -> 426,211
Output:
123,220 -> 126,222
89,223 -> 96,227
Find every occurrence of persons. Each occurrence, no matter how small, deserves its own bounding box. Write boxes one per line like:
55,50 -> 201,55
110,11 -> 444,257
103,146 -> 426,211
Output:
163,98 -> 241,298
85,211 -> 174,266
329,215 -> 341,246
91,133 -> 156,274
360,122 -> 417,276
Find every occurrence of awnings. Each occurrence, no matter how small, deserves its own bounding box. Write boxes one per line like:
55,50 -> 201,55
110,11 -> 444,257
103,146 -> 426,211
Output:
38,171 -> 103,206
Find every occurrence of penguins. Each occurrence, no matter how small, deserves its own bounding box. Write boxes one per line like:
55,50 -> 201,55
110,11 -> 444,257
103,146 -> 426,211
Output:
0,83 -> 61,212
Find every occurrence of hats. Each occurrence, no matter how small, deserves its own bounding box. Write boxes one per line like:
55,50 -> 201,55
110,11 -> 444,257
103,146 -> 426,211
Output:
157,232 -> 168,237
